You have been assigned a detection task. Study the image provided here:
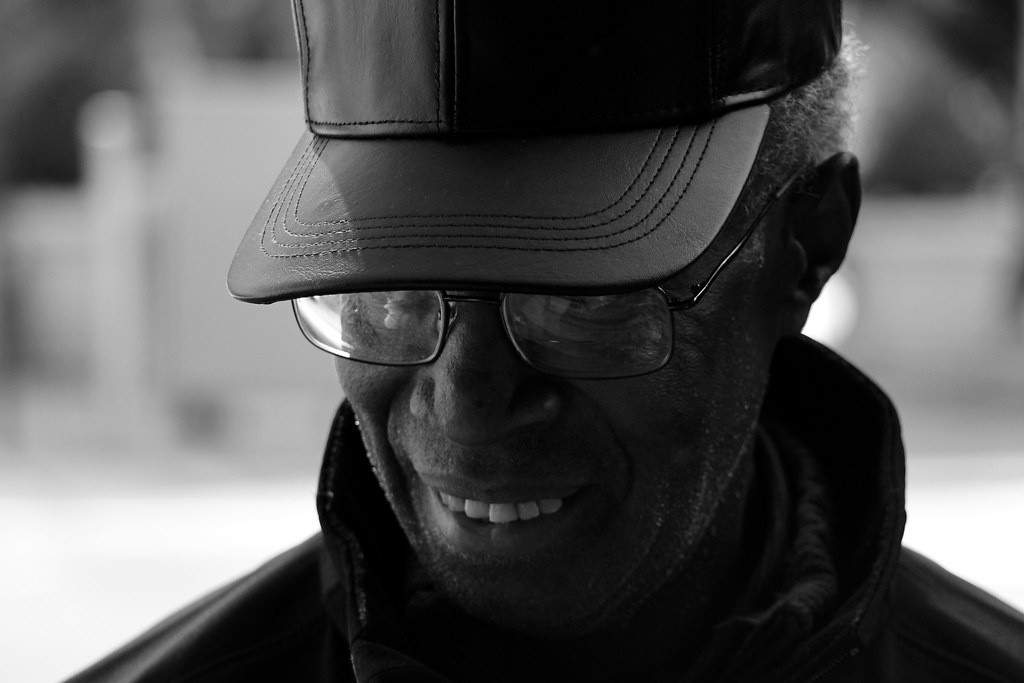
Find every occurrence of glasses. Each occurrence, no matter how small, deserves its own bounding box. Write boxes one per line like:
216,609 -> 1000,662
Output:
291,164 -> 815,382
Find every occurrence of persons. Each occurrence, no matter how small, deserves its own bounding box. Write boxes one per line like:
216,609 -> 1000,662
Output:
51,1 -> 1024,683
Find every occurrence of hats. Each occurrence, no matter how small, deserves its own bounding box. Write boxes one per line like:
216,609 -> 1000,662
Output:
225,0 -> 842,303
